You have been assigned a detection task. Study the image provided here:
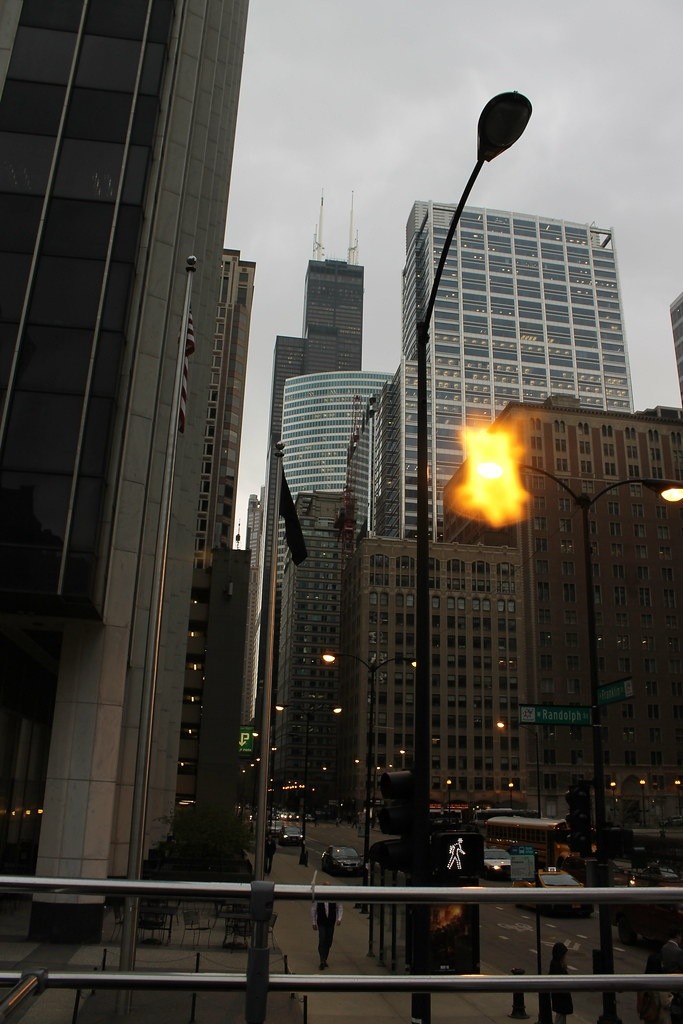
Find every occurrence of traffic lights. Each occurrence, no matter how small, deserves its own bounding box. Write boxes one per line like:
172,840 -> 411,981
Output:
375,769 -> 411,874
437,835 -> 484,877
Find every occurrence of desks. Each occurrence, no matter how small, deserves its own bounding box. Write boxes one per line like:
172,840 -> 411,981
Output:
139,905 -> 178,939
224,899 -> 249,912
218,911 -> 253,950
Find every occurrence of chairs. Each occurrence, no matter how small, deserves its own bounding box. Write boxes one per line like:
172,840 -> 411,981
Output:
109,896 -> 278,954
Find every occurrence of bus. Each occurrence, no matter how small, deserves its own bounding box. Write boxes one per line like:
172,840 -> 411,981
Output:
430,809 -> 463,824
484,815 -> 574,869
473,808 -> 513,823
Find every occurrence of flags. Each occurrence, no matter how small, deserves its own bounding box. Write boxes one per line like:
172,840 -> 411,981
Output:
278,464 -> 308,566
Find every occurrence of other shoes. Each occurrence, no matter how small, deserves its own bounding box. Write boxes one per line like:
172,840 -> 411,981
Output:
319,961 -> 329,970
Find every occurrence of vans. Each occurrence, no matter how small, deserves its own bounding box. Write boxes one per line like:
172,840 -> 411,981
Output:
563,855 -> 613,888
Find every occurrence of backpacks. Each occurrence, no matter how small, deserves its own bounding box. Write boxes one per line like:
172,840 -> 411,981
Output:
637,990 -> 660,1023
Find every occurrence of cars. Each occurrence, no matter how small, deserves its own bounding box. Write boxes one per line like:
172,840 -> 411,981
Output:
659,815 -> 683,827
608,883 -> 683,946
268,820 -> 282,835
484,848 -> 513,878
320,844 -> 364,877
512,866 -> 595,917
279,824 -> 304,846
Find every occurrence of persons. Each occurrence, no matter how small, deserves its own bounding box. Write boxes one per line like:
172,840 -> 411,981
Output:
548,943 -> 574,1024
311,881 -> 344,971
263,834 -> 277,876
638,929 -> 683,1024
313,811 -> 359,829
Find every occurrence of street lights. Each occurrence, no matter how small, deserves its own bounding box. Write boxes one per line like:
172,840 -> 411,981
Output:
508,783 -> 514,808
410,89 -> 538,1024
446,779 -> 452,823
274,703 -> 343,866
610,781 -> 617,819
639,779 -> 646,826
471,457 -> 683,829
674,779 -> 681,815
319,650 -> 417,914
